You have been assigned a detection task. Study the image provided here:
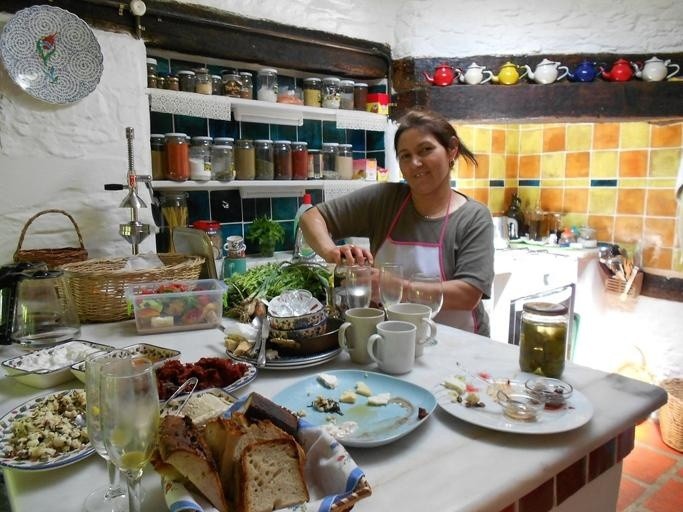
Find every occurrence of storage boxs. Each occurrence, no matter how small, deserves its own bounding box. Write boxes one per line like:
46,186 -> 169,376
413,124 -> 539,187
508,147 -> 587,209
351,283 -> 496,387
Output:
126,278 -> 229,333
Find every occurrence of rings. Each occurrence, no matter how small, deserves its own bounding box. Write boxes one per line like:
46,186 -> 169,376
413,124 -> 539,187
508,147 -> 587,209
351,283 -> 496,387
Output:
349,243 -> 356,249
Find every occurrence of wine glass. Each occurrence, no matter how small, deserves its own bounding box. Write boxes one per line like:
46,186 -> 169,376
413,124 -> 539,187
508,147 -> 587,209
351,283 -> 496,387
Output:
343,263 -> 443,345
85,348 -> 141,511
100,358 -> 161,511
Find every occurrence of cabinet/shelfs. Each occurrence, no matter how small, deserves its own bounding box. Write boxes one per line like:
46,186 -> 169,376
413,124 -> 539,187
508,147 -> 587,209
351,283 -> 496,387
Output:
124,11 -> 391,263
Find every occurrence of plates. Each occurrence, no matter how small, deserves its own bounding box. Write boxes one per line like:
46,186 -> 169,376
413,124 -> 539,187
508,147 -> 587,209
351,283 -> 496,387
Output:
0,390 -> 95,470
223,349 -> 345,370
270,369 -> 438,448
162,358 -> 256,402
429,366 -> 593,435
0,4 -> 104,104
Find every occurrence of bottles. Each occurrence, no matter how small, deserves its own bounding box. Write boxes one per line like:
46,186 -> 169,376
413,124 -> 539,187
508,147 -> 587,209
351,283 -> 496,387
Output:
529,200 -> 543,241
156,192 -> 192,254
549,212 -> 563,242
193,220 -> 224,260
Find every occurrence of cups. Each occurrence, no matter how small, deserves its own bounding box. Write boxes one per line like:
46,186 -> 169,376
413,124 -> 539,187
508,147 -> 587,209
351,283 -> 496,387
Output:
221,235 -> 248,279
524,377 -> 573,410
488,377 -> 523,401
495,388 -> 547,420
340,303 -> 436,373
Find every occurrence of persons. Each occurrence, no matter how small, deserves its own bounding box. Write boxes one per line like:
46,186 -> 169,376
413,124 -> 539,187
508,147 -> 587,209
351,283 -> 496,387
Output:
300,108 -> 494,338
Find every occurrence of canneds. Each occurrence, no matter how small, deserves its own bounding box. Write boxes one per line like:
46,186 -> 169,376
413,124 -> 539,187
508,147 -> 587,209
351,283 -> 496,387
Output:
147,58 -> 278,103
150,132 -> 354,180
322,78 -> 340,108
519,300 -> 569,380
302,78 -> 323,107
354,83 -> 368,110
339,80 -> 355,109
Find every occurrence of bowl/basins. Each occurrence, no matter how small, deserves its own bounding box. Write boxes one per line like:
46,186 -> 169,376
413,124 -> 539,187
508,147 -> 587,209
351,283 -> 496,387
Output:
270,315 -> 329,340
269,304 -> 327,330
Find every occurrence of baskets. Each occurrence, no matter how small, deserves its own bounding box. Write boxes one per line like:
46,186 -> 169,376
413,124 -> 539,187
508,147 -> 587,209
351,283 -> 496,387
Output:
330,476 -> 371,512
12,209 -> 88,264
605,272 -> 643,296
59,254 -> 204,322
659,378 -> 683,452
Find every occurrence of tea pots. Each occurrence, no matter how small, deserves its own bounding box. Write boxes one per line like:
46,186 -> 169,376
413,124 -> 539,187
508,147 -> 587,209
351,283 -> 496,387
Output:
421,56 -> 679,86
0,270 -> 83,348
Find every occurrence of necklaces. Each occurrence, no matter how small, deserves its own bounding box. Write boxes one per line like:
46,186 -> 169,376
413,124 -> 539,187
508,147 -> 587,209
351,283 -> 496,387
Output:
413,196 -> 451,219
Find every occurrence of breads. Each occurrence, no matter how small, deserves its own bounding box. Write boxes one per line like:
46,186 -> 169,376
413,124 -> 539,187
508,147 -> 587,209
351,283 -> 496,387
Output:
146,392 -> 309,511
